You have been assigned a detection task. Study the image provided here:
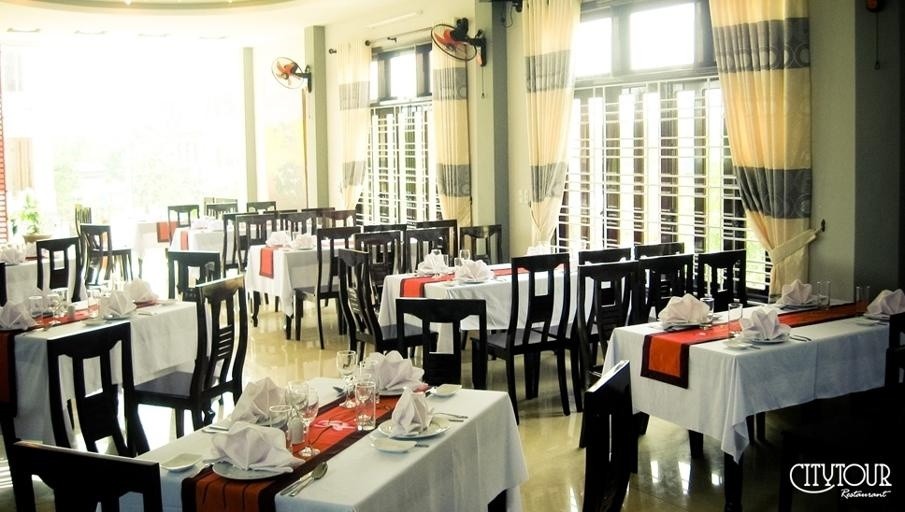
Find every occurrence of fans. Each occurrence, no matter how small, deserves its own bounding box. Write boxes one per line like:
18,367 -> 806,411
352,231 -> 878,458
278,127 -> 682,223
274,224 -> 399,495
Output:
267,56 -> 314,94
427,14 -> 490,69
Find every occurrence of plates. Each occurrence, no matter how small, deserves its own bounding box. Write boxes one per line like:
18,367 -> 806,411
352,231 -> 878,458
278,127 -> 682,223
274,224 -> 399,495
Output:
463,280 -> 484,284
786,303 -> 818,308
104,311 -> 135,319
266,243 -> 282,248
132,299 -> 152,304
431,383 -> 462,399
160,452 -> 202,473
211,461 -> 285,482
297,244 -> 316,250
662,315 -> 721,327
380,383 -> 433,397
371,436 -> 416,454
723,339 -> 753,350
751,336 -> 790,343
378,416 -> 450,439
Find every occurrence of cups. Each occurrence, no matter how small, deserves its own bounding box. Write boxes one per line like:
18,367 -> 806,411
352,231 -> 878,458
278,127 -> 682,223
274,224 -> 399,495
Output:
701,298 -> 714,330
817,280 -> 831,311
353,381 -> 376,431
360,360 -> 381,404
268,405 -> 293,450
728,303 -> 744,340
856,284 -> 870,314
28,286 -> 101,332
431,248 -> 470,280
282,230 -> 300,248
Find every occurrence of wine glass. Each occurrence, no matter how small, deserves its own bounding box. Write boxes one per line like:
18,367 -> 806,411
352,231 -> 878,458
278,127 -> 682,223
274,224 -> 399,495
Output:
337,350 -> 357,408
285,381 -> 322,458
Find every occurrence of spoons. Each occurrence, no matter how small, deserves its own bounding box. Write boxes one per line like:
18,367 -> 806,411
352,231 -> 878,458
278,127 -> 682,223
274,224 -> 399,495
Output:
332,385 -> 343,392
280,461 -> 328,496
